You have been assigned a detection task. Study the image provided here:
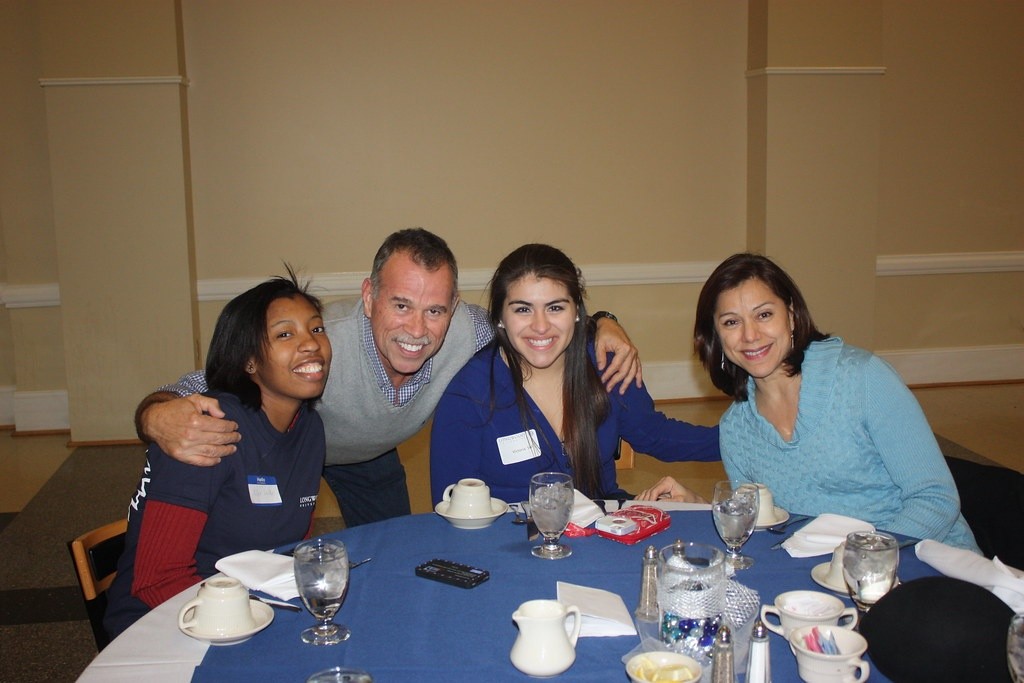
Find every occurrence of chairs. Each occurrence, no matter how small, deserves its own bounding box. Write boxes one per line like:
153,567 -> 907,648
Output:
66,518 -> 127,653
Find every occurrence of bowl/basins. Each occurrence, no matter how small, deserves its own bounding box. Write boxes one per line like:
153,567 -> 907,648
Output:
435,496 -> 508,530
625,651 -> 702,683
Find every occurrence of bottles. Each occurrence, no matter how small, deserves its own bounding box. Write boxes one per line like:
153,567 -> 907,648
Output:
711,627 -> 735,683
671,538 -> 686,561
744,621 -> 771,683
634,546 -> 658,624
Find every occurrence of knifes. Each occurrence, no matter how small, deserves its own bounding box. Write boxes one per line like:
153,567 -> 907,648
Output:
200,581 -> 302,612
770,537 -> 792,550
521,501 -> 539,541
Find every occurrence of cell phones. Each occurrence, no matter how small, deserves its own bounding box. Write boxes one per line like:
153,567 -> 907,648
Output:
415,559 -> 490,588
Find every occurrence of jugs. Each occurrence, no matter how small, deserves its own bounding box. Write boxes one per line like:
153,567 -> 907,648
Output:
510,599 -> 581,678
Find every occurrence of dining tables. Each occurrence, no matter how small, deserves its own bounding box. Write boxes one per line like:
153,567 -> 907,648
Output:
72,502 -> 1024,683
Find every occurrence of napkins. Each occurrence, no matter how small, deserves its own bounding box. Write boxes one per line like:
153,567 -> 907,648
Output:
556,580 -> 637,638
214,549 -> 303,602
570,488 -> 606,526
781,512 -> 876,560
913,538 -> 1024,615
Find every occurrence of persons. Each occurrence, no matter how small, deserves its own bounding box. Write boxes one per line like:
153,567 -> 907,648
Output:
429,243 -> 722,500
105,279 -> 333,638
133,229 -> 643,528
633,254 -> 985,557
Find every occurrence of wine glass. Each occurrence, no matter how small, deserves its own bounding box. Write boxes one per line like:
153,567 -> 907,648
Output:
529,472 -> 574,560
293,538 -> 352,646
712,481 -> 760,570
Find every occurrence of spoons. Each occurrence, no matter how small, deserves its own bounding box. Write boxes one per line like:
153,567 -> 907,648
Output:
509,504 -> 525,524
767,516 -> 809,534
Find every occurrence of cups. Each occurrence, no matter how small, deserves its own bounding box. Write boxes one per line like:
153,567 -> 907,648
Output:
824,541 -> 847,589
442,479 -> 492,518
658,542 -> 726,666
760,590 -> 858,642
843,531 -> 900,610
178,577 -> 256,635
753,483 -> 777,524
307,666 -> 373,683
790,625 -> 870,683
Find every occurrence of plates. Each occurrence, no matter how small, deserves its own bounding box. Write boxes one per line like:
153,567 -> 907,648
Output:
753,507 -> 789,531
810,562 -> 849,599
181,600 -> 274,646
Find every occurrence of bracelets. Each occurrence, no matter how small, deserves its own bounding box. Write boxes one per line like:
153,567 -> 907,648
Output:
592,311 -> 618,322
